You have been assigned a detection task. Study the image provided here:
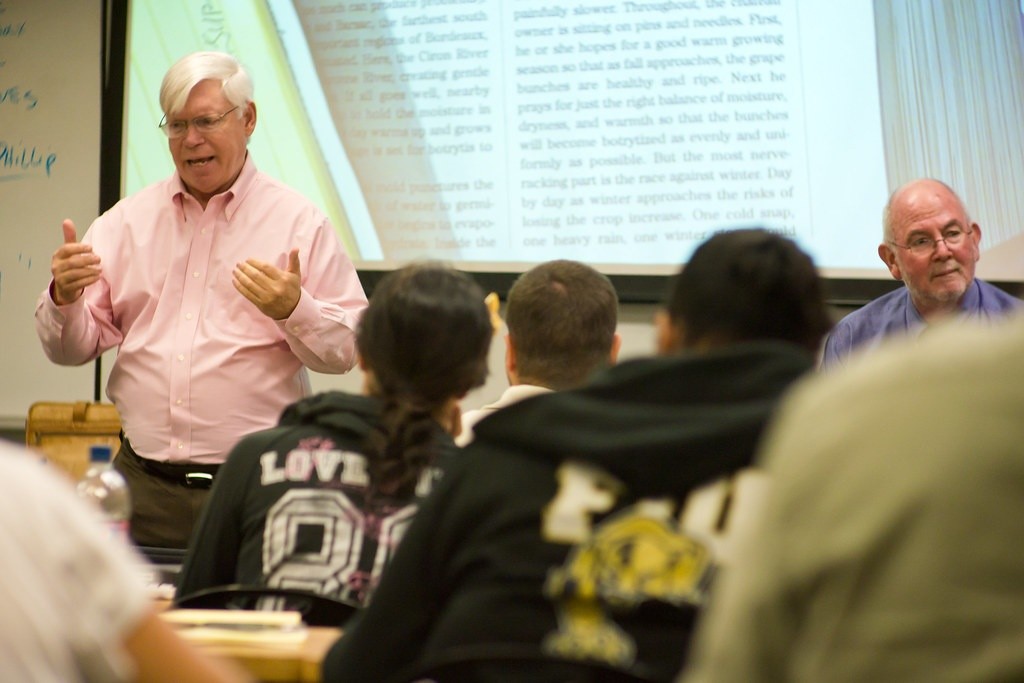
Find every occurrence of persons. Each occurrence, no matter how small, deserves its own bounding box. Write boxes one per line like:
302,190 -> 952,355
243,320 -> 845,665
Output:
33,48 -> 370,550
0,439 -> 231,683
453,258 -> 623,450
171,261 -> 498,630
818,178 -> 1022,373
316,229 -> 836,683
677,300 -> 1023,683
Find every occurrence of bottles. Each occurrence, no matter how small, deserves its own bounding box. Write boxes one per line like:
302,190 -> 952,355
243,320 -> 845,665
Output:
78,447 -> 131,537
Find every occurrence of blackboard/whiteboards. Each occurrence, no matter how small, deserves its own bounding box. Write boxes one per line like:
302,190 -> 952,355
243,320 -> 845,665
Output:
0,0 -> 114,435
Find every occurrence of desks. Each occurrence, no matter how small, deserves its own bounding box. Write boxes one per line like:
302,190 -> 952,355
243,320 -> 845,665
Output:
186,626 -> 344,683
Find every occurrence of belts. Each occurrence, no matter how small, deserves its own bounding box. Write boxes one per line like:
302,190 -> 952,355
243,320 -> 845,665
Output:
124,439 -> 220,489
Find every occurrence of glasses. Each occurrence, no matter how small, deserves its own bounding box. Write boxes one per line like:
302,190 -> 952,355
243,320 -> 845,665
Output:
158,105 -> 239,139
887,224 -> 973,254
